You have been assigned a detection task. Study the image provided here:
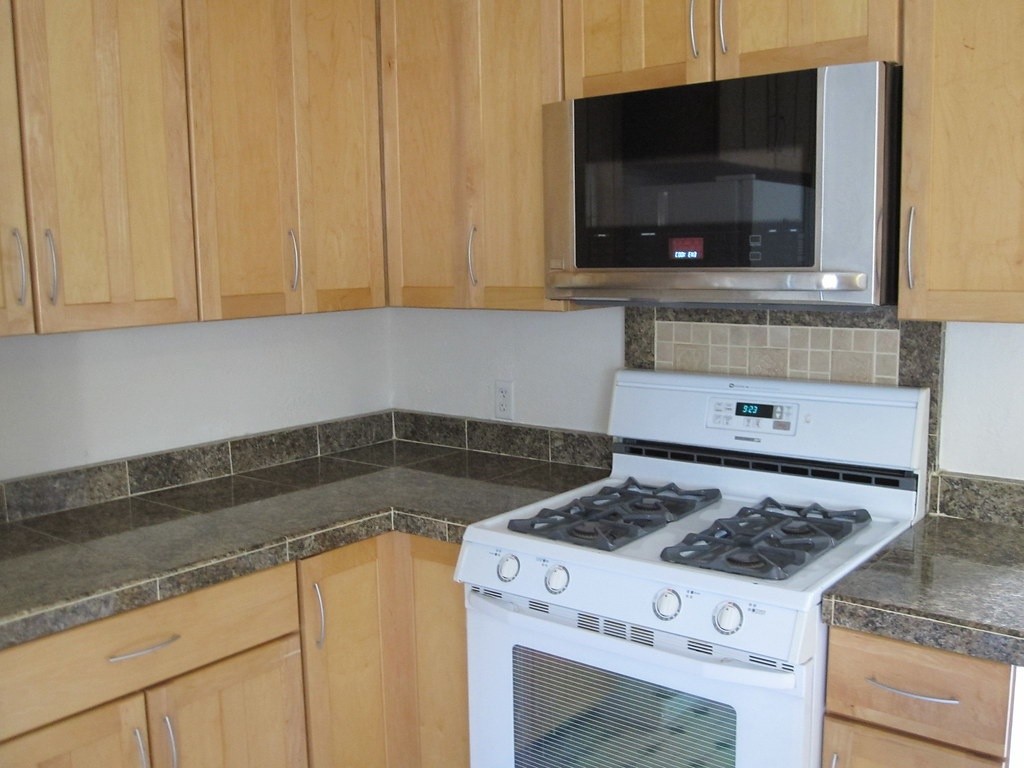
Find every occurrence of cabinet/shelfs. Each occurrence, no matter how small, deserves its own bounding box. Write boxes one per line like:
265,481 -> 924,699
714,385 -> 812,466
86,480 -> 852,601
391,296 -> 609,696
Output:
822,626 -> 1024,768
563,0 -> 904,103
183,0 -> 386,325
896,0 -> 1024,322
0,561 -> 310,768
298,532 -> 418,768
0,0 -> 197,336
379,0 -> 588,311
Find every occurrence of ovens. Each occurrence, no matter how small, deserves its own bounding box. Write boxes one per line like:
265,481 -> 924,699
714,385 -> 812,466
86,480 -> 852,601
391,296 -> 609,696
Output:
465,583 -> 827,767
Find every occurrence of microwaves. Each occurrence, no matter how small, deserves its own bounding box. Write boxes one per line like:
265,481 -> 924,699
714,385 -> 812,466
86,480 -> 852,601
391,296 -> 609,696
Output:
544,61 -> 898,308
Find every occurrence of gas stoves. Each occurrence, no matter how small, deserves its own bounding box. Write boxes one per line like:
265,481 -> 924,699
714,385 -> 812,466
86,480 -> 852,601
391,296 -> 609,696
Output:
453,370 -> 930,662
